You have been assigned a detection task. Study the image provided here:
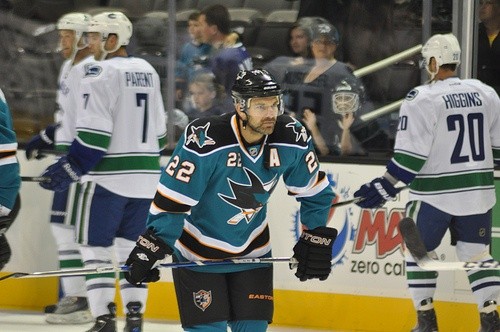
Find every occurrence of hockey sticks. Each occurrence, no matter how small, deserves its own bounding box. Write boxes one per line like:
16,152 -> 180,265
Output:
397,217 -> 500,272
331,187 -> 412,212
21,173 -> 56,181
0,255 -> 295,284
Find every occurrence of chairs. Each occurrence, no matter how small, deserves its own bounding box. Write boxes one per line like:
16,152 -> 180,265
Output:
0,0 -> 301,153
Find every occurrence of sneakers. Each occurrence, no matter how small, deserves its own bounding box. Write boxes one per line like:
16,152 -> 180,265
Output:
42,296 -> 94,326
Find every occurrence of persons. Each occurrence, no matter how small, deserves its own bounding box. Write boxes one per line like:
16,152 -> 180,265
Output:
122,67 -> 339,332
1,87 -> 24,274
279,16 -> 377,158
354,32 -> 499,331
38,10 -> 169,331
27,12 -> 93,325
472,0 -> 500,97
175,2 -> 253,139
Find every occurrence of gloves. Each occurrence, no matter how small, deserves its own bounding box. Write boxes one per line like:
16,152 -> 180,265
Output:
290,225 -> 338,282
122,229 -> 174,287
0,191 -> 21,272
24,124 -> 62,162
353,156 -> 422,211
37,134 -> 108,192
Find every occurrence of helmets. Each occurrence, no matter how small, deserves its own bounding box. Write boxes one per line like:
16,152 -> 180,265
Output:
420,32 -> 461,77
230,69 -> 284,117
56,12 -> 92,50
310,23 -> 340,46
328,77 -> 367,115
77,11 -> 133,53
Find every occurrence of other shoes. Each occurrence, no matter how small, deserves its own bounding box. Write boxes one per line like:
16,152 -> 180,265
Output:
409,307 -> 439,332
85,301 -> 118,332
122,300 -> 144,332
477,299 -> 500,332
